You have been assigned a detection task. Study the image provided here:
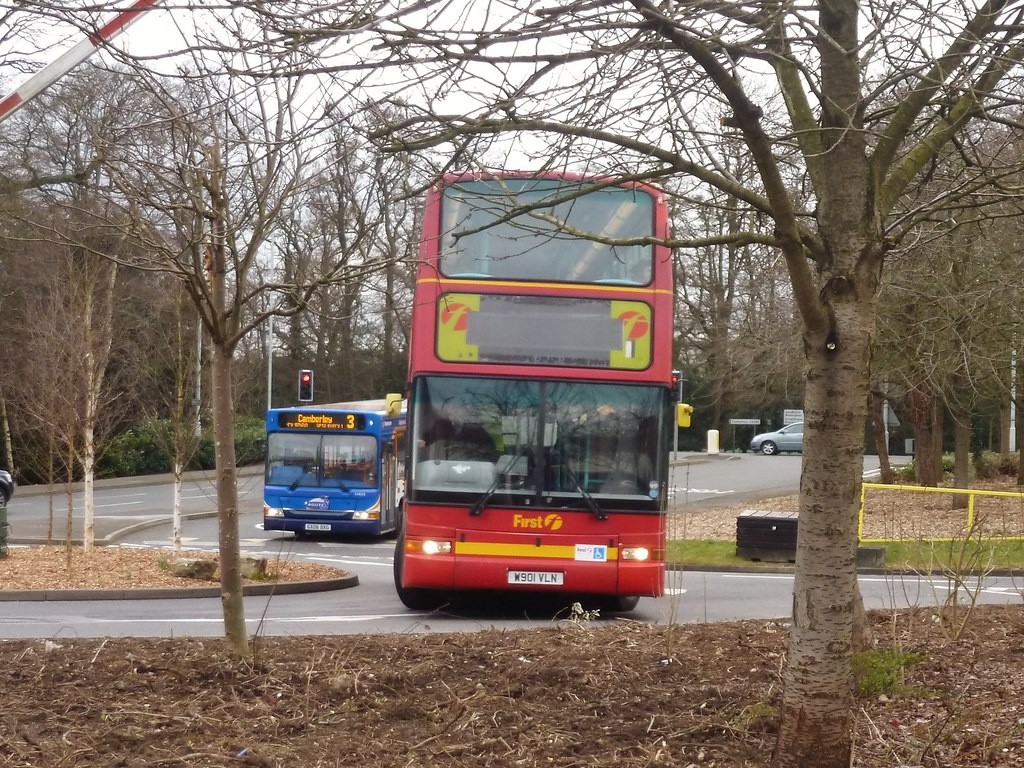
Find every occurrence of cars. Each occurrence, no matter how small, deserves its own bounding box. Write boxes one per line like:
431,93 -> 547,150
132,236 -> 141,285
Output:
750,421 -> 804,455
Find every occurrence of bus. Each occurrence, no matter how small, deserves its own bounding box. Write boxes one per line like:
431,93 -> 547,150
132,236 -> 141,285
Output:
385,168 -> 695,614
254,399 -> 408,540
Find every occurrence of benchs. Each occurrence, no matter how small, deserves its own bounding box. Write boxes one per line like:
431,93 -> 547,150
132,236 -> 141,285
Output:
736,510 -> 799,562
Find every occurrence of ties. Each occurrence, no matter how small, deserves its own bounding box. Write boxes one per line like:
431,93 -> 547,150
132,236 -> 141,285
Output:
454,420 -> 460,432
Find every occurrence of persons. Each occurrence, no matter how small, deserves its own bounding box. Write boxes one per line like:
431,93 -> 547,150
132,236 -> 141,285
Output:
630,258 -> 652,284
554,407 -> 604,452
328,455 -> 347,473
417,401 -> 496,461
440,240 -> 465,275
356,450 -> 373,475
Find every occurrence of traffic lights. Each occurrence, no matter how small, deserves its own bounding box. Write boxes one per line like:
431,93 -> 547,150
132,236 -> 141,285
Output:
298,370 -> 314,401
672,370 -> 681,392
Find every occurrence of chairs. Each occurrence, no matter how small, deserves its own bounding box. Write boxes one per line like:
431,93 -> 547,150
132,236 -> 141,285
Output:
327,462 -> 372,482
444,394 -> 619,494
